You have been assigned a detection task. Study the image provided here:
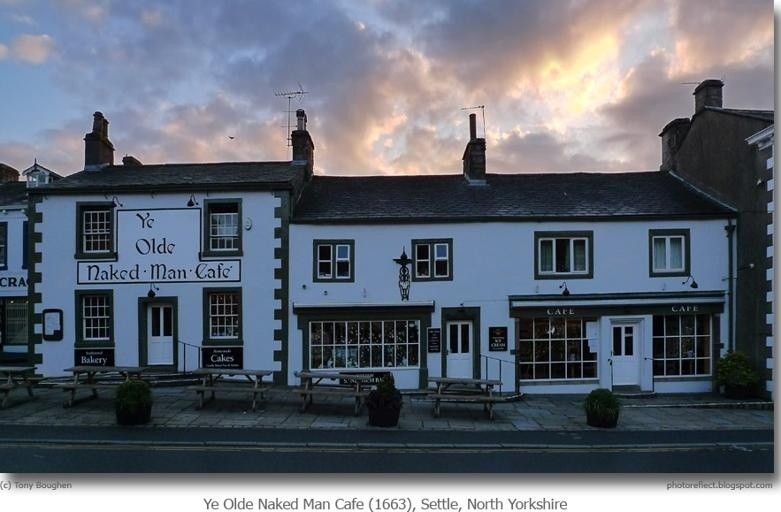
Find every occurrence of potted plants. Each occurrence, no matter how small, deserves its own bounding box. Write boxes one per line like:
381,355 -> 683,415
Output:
362,373 -> 406,427
113,379 -> 155,425
713,349 -> 763,399
585,387 -> 623,429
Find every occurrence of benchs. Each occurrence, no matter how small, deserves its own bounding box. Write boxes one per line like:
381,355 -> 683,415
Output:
0,376 -> 159,407
187,377 -> 272,412
293,383 -> 372,416
427,386 -> 507,419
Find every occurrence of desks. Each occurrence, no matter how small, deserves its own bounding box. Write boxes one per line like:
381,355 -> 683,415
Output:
294,370 -> 373,415
0,365 -> 39,407
426,374 -> 505,419
63,364 -> 151,406
190,368 -> 274,411
